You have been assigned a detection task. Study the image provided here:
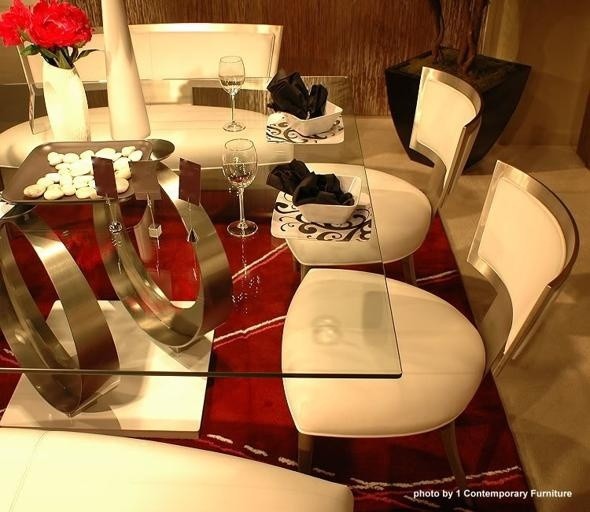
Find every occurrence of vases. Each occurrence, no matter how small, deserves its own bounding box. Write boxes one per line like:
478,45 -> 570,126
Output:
387,45 -> 531,170
41,58 -> 92,142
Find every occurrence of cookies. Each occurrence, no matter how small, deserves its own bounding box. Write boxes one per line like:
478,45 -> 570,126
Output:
24,146 -> 142,200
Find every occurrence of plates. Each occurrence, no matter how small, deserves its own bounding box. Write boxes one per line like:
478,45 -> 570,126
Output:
0,297 -> 216,437
0,139 -> 153,204
264,113 -> 344,145
270,190 -> 373,242
147,137 -> 175,162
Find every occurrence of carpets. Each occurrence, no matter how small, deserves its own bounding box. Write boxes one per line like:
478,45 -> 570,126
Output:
0,189 -> 537,511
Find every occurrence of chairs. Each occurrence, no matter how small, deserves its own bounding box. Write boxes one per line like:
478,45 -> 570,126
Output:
1,427 -> 355,512
276,67 -> 580,489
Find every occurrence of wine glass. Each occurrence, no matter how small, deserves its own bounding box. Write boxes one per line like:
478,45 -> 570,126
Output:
221,138 -> 260,238
219,55 -> 247,132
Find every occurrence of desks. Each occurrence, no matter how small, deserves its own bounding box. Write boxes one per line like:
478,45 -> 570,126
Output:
0,74 -> 402,442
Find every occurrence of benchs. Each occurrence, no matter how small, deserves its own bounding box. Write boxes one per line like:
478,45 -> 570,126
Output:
0,21 -> 293,172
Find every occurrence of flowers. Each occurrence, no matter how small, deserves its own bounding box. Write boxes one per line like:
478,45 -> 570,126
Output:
0,0 -> 99,68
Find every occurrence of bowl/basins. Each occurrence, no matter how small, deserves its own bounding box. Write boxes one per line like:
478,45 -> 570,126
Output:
284,99 -> 343,137
296,175 -> 361,223
